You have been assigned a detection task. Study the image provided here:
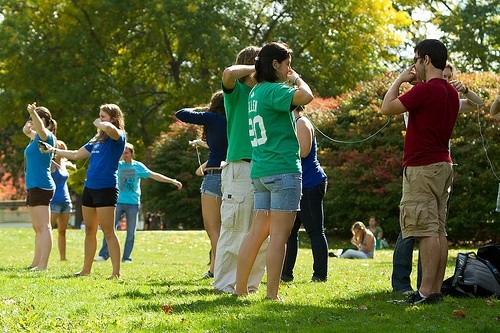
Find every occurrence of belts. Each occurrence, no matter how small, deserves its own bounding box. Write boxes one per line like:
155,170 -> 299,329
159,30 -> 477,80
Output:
242,158 -> 251,163
204,169 -> 222,175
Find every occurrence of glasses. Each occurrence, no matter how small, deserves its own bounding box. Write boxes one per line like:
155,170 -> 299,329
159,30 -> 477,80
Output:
414,57 -> 421,63
27,116 -> 33,121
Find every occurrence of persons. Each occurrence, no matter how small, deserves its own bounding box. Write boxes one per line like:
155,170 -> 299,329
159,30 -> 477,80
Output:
176,43 -> 328,300
382,39 -> 483,304
93,144 -> 182,262
23,103 -> 76,271
50,140 -> 76,261
41,104 -> 127,279
342,215 -> 383,259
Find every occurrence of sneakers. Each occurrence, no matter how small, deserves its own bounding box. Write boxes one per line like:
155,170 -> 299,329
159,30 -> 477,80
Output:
397,291 -> 443,305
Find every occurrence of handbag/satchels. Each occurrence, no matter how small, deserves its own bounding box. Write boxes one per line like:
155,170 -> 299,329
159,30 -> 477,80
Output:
441,252 -> 500,298
377,237 -> 389,250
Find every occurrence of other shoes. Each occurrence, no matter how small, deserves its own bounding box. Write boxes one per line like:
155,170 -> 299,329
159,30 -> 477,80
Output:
94,256 -> 107,261
329,252 -> 337,258
201,271 -> 214,280
121,259 -> 133,263
403,290 -> 416,295
